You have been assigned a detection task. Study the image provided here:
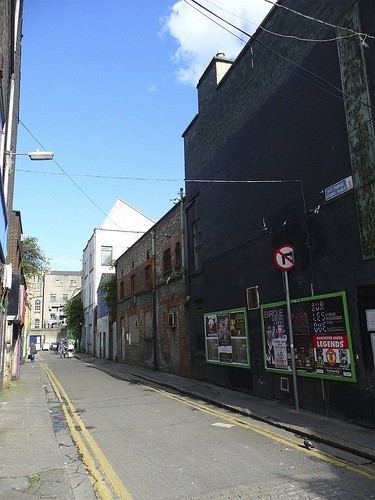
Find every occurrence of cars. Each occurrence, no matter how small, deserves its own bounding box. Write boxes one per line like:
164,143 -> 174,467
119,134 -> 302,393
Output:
42,342 -> 57,351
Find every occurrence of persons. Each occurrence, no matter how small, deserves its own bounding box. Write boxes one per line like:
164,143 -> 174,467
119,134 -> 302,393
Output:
30,341 -> 36,361
58,341 -> 67,359
264,317 -> 275,368
